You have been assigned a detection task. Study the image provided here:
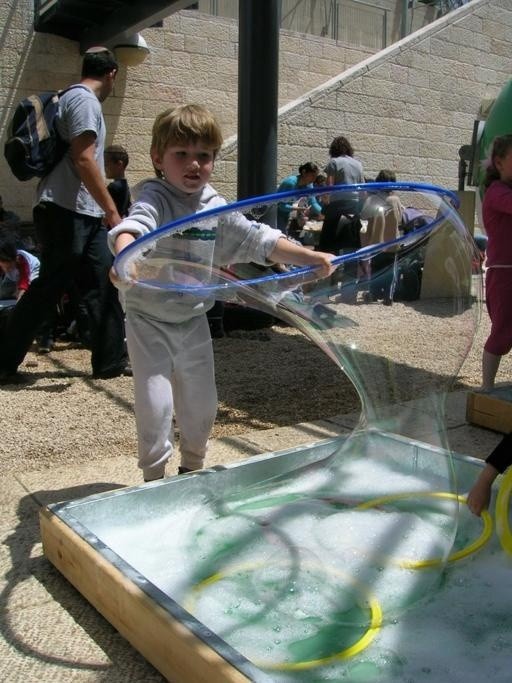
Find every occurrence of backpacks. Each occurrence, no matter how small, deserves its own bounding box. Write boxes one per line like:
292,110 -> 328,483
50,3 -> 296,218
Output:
4,84 -> 94,182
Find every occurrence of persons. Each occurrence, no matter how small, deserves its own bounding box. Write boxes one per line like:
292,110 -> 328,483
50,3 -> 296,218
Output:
104,144 -> 131,219
467,429 -> 512,517
0,240 -> 53,352
0,45 -> 135,385
481,134 -> 512,388
107,103 -> 338,482
277,136 -> 433,305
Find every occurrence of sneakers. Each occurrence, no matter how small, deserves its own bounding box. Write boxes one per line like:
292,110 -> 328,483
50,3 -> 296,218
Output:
305,294 -> 394,307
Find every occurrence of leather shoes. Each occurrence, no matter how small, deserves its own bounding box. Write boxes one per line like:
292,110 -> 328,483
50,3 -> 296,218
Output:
108,362 -> 134,377
